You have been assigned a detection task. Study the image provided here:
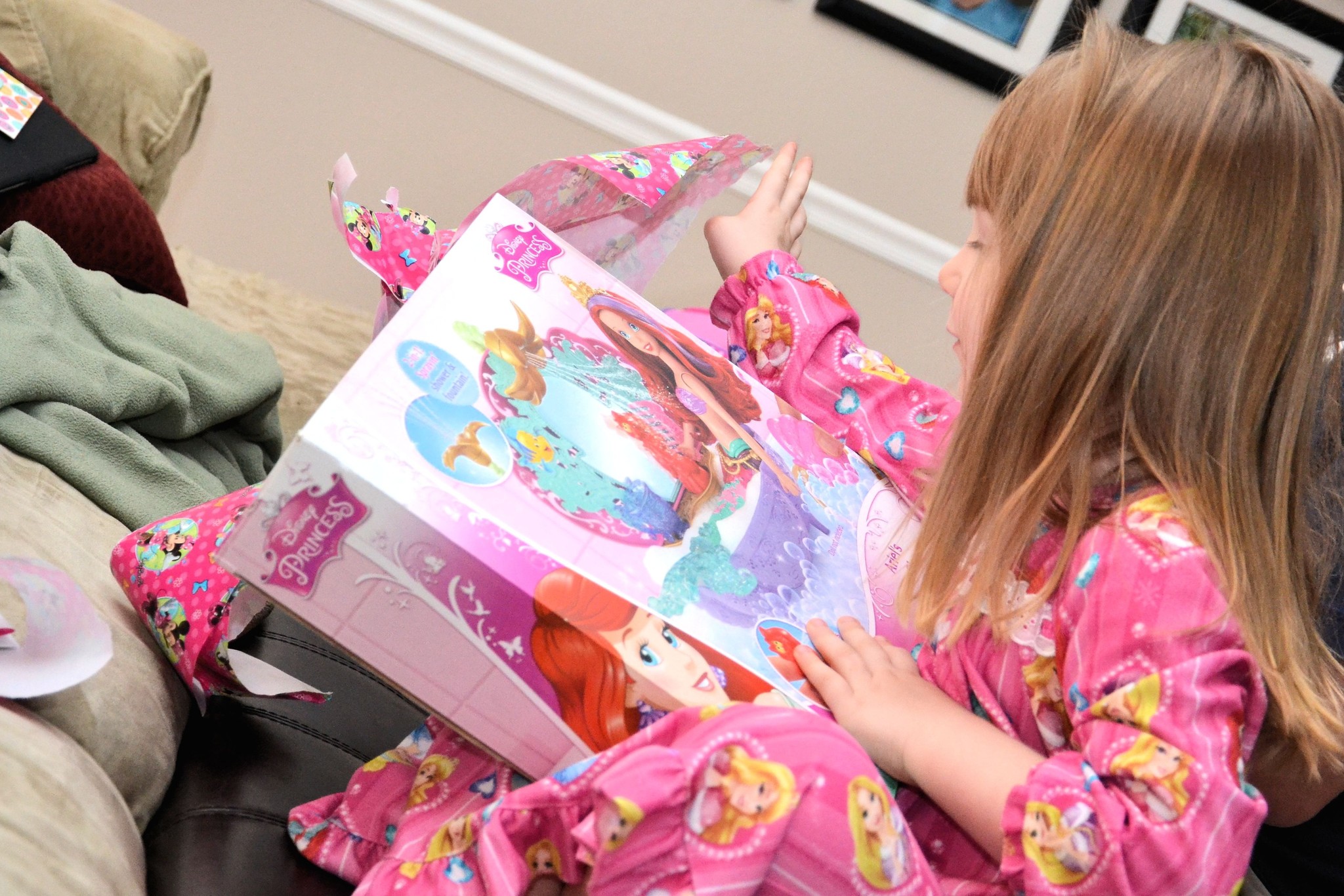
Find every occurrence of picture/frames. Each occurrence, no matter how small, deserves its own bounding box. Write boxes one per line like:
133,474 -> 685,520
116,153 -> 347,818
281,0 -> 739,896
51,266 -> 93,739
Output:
814,0 -> 1098,98
1120,0 -> 1343,106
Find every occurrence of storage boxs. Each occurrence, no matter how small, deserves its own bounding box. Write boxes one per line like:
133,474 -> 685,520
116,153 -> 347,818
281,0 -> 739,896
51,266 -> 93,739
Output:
211,191 -> 942,799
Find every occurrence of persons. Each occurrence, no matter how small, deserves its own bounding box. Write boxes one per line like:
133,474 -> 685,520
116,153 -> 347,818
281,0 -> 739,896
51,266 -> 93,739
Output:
291,22 -> 1344,896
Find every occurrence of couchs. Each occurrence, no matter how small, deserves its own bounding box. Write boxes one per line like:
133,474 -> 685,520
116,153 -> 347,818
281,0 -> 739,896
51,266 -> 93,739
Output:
0,0 -> 1343,896
